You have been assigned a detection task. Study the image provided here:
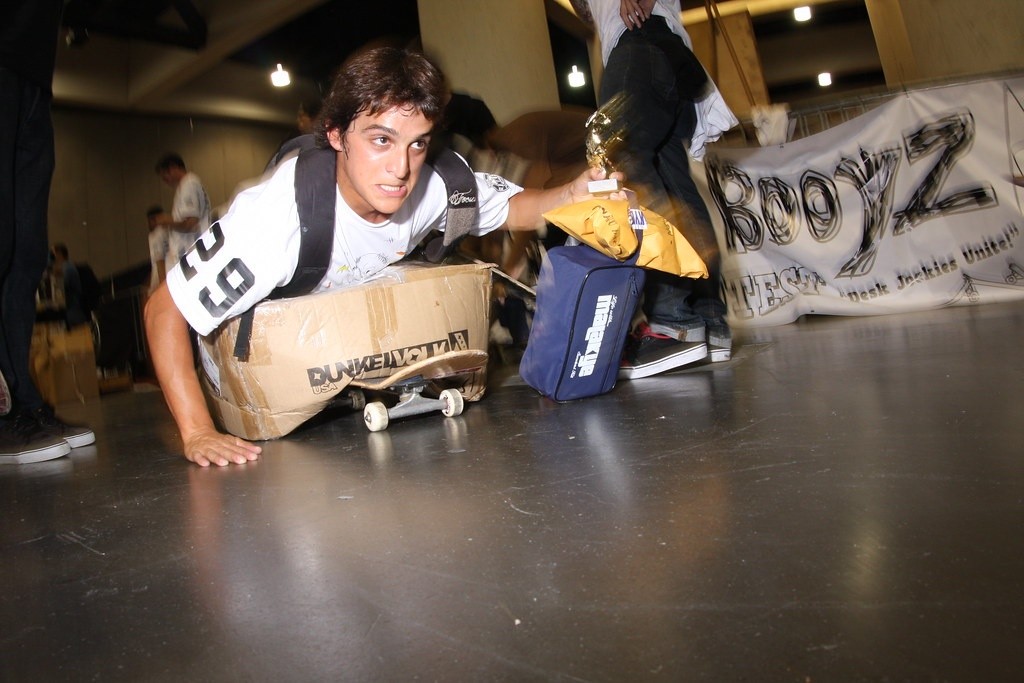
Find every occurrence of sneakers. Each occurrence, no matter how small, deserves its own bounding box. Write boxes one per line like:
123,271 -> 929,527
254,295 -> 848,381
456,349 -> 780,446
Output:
0,411 -> 71,464
617,321 -> 708,380
706,343 -> 731,363
44,415 -> 95,448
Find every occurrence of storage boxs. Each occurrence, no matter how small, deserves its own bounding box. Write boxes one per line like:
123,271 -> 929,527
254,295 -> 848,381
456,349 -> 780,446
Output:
195,262 -> 497,443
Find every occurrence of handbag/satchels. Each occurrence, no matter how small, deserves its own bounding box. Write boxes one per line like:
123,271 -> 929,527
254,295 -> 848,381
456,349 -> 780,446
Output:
519,188 -> 647,403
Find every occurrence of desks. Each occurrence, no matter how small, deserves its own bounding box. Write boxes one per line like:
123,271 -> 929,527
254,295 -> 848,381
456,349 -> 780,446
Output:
31,304 -> 103,404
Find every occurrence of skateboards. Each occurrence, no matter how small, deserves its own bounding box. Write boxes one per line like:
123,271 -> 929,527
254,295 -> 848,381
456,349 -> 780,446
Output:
324,348 -> 491,433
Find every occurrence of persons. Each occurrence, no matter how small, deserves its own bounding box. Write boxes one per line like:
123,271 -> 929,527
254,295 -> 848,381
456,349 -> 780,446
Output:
144,48 -> 627,467
36,59 -> 496,386
588,0 -> 740,379
0,0 -> 95,464
